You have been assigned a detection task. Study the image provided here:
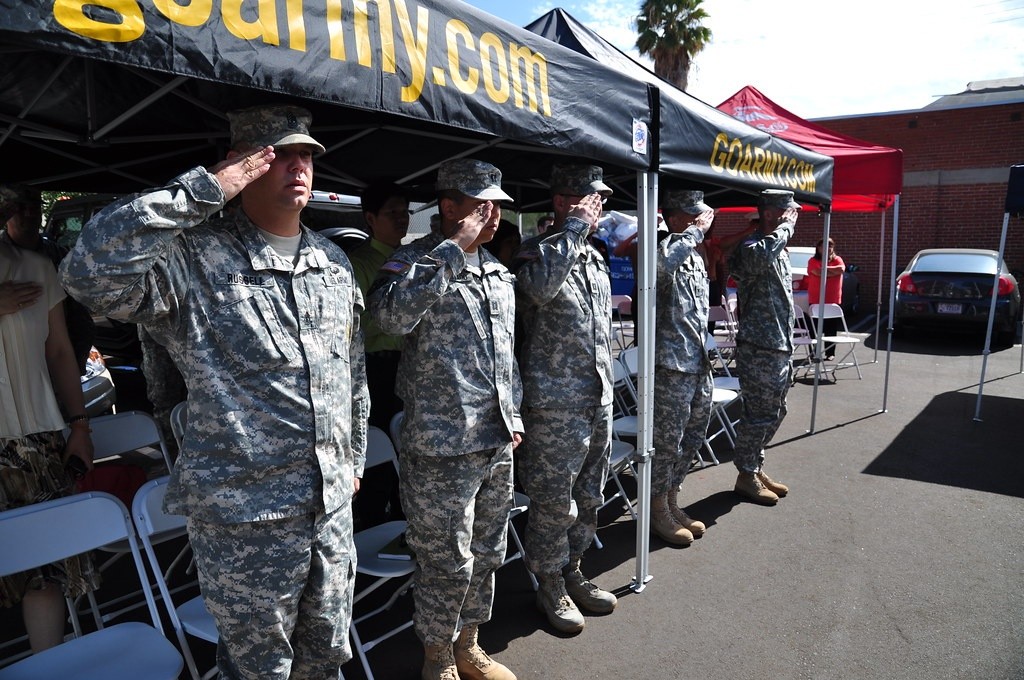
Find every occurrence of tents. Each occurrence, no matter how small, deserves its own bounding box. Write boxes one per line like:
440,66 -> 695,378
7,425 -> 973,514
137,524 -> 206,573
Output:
1,0 -> 657,594
521,9 -> 835,587
973,163 -> 1024,423
714,87 -> 902,413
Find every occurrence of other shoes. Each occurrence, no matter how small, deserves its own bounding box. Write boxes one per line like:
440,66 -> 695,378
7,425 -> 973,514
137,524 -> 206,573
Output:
827,356 -> 835,361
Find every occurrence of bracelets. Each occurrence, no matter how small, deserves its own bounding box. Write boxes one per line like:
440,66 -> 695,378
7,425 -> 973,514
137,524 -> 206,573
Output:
68,414 -> 89,431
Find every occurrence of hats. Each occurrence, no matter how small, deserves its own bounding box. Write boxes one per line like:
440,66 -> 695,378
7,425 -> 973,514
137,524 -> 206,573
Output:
549,163 -> 614,196
663,190 -> 714,215
435,158 -> 514,203
227,103 -> 326,154
757,189 -> 802,210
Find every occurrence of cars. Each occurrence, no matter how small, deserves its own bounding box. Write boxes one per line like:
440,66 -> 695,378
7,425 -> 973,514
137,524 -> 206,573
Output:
895,247 -> 1022,352
781,245 -> 816,329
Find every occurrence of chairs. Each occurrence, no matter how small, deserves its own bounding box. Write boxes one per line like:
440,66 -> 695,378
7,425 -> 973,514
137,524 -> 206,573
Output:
0,295 -> 863,680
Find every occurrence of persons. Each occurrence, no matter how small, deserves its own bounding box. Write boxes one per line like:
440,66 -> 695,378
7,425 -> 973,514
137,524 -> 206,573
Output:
807,237 -> 846,362
613,214 -> 762,347
55,102 -> 370,680
725,188 -> 802,505
364,156 -> 527,680
341,174 -> 608,531
0,178 -> 190,658
650,189 -> 714,547
515,159 -> 620,635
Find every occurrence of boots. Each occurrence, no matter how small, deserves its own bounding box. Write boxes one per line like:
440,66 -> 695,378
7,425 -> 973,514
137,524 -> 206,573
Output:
454,623 -> 517,680
536,569 -> 585,632
758,470 -> 789,495
563,553 -> 617,613
735,470 -> 779,503
422,642 -> 461,680
668,491 -> 706,535
651,491 -> 694,544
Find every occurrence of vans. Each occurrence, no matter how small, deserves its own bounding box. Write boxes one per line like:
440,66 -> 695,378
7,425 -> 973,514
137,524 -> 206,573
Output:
44,194 -> 120,248
